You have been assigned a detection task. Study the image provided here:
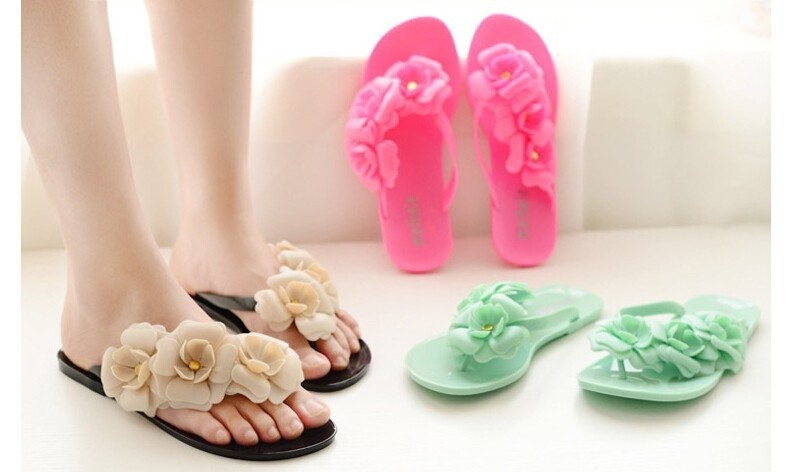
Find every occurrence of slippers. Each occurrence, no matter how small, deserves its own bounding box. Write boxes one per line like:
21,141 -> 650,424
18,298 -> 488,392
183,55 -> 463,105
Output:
189,240 -> 372,393
404,280 -> 604,397
466,12 -> 559,268
57,316 -> 337,461
575,290 -> 762,404
346,15 -> 463,275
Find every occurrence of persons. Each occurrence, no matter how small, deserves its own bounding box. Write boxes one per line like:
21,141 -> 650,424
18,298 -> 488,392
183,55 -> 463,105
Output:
21,0 -> 374,463
